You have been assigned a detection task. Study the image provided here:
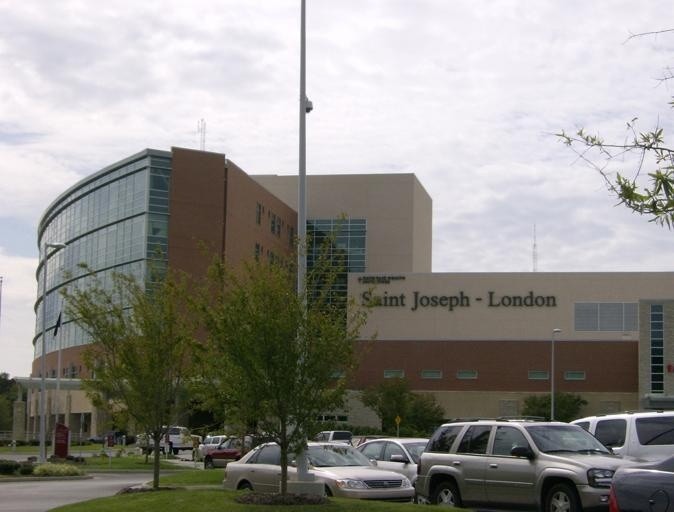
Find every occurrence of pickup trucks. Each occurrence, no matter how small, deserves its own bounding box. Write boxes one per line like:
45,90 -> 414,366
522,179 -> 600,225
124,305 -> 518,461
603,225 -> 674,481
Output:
136,427 -> 204,455
312,430 -> 354,443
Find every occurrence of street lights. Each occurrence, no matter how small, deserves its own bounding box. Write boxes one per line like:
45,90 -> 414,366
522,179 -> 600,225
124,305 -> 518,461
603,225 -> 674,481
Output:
551,329 -> 562,421
33,242 -> 67,464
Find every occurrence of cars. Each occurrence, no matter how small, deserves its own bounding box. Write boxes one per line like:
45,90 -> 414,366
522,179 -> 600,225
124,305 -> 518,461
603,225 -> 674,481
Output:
350,436 -> 392,448
204,434 -> 261,468
223,442 -> 416,503
355,437 -> 430,488
609,456 -> 674,512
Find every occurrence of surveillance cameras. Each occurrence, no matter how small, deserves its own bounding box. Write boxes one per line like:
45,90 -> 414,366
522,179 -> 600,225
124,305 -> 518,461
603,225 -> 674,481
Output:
306,96 -> 313,114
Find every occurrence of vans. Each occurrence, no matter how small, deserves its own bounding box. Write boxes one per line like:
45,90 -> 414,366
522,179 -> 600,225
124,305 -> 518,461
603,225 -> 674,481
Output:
563,410 -> 674,461
192,435 -> 238,461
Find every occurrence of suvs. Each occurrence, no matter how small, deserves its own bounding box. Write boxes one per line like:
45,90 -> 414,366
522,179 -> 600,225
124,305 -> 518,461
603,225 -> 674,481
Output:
415,416 -> 644,512
